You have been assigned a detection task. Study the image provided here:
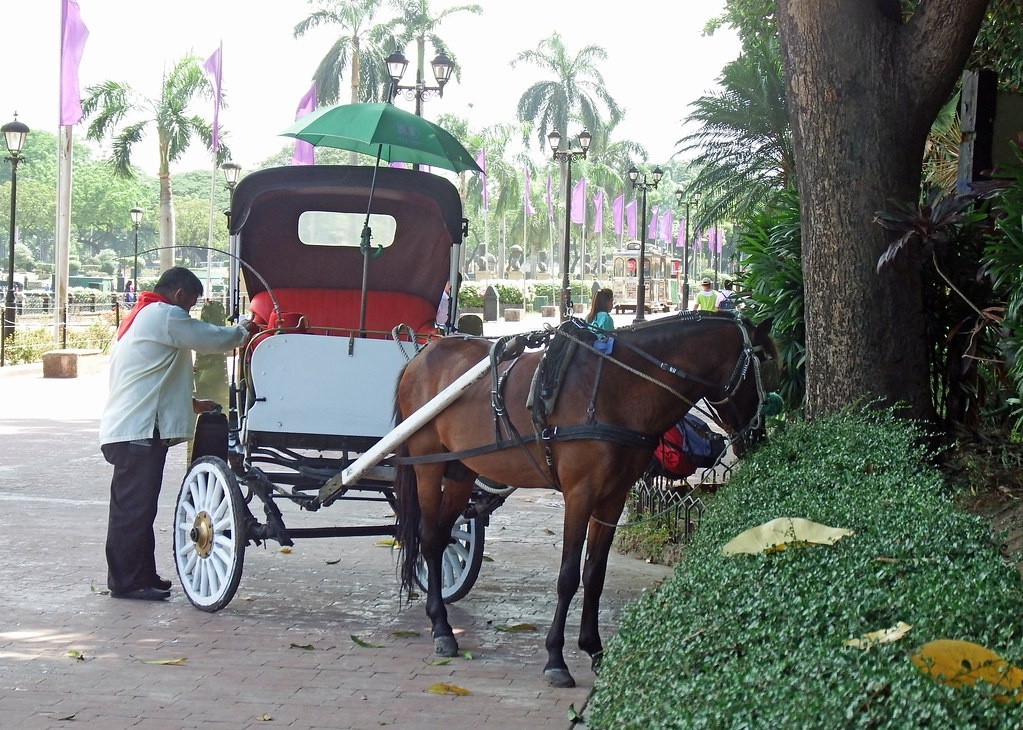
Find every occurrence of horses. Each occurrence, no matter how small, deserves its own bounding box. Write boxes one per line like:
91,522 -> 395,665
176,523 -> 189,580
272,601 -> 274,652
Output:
390,308 -> 781,691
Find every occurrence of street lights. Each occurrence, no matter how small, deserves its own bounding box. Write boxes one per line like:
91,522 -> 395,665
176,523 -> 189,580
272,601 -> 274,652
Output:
383,46 -> 455,171
0,110 -> 30,341
129,202 -> 143,295
675,188 -> 702,311
548,126 -> 593,322
628,165 -> 664,324
218,162 -> 242,210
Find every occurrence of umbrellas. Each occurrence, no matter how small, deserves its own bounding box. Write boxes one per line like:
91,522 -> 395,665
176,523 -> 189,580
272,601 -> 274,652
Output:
275,102 -> 487,259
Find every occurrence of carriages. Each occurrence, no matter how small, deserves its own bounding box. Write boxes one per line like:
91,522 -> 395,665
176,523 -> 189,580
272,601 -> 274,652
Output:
173,165 -> 784,687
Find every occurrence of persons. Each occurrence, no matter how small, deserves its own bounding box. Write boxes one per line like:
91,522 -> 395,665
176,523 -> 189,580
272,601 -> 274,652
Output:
694,277 -> 719,311
435,271 -> 462,333
585,288 -> 615,340
715,279 -> 741,310
124,280 -> 134,310
98,265 -> 257,601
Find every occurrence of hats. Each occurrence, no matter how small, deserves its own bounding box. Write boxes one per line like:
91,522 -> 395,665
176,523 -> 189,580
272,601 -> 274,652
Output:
701,278 -> 711,284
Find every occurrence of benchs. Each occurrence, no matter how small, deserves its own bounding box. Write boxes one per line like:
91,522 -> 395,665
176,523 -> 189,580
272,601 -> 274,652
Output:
240,289 -> 439,350
614,303 -> 652,314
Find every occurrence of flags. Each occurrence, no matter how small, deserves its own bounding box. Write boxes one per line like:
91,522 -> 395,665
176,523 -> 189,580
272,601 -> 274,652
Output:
388,162 -> 406,168
612,195 -> 623,234
546,175 -> 554,224
60,1 -> 89,126
626,199 -> 637,237
291,85 -> 316,166
570,178 -> 586,224
676,219 -> 686,248
707,225 -> 723,253
649,208 -> 672,243
201,45 -> 222,152
690,222 -> 703,250
593,190 -> 603,232
525,166 -> 536,216
475,148 -> 487,209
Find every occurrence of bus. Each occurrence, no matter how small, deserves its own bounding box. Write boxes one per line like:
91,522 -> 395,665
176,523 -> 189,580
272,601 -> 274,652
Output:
612,241 -> 673,314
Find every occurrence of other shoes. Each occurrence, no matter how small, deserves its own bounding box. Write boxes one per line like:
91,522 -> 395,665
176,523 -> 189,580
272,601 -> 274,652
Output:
110,577 -> 172,600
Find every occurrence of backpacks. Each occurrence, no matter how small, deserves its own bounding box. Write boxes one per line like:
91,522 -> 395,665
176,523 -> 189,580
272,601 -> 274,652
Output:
660,411 -> 727,469
719,292 -> 735,309
